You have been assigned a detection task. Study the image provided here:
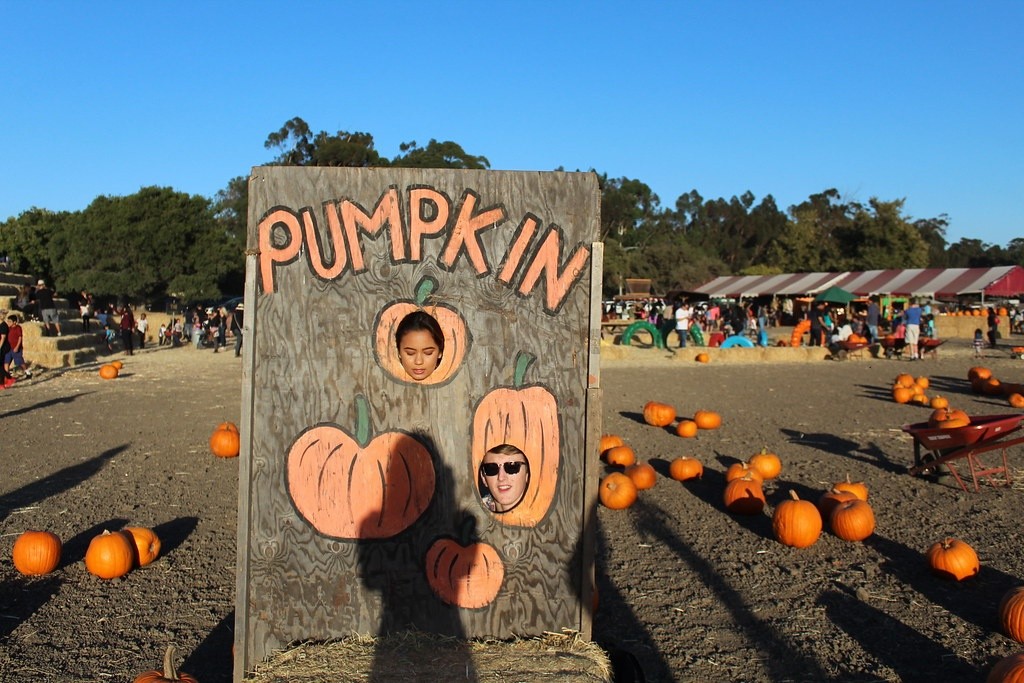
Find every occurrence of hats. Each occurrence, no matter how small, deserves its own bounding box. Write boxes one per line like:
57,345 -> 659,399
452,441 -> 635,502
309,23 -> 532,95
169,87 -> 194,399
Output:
235,303 -> 244,310
38,280 -> 45,286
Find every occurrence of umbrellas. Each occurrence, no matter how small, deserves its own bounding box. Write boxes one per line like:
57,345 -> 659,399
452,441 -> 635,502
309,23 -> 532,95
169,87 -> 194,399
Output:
813,285 -> 858,304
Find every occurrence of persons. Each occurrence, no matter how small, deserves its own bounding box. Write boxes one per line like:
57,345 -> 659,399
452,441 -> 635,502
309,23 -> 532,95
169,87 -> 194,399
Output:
480,444 -> 530,514
0,309 -> 16,389
4,315 -> 31,379
601,295 -> 1024,359
0,249 -> 246,356
395,311 -> 445,380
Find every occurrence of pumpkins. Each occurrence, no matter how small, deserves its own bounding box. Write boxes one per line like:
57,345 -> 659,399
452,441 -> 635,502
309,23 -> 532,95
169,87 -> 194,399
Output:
99,361 -> 122,379
85,527 -> 162,579
12,529 -> 61,576
209,420 -> 240,458
885,334 -> 895,338
847,334 -> 867,343
984,653 -> 1024,683
892,367 -> 1024,428
594,582 -> 599,615
596,402 -> 875,549
998,585 -> 1024,644
130,645 -> 200,683
698,353 -> 708,363
956,307 -> 1007,316
926,537 -> 980,586
1012,346 -> 1024,353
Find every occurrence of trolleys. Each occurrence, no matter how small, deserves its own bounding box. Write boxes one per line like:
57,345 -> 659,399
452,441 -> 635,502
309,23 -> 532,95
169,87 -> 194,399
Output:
878,337 -> 909,360
901,414 -> 1024,494
917,338 -> 949,360
834,340 -> 881,361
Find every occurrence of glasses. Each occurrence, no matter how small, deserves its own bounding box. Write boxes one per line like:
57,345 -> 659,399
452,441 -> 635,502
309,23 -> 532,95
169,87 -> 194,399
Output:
481,461 -> 528,476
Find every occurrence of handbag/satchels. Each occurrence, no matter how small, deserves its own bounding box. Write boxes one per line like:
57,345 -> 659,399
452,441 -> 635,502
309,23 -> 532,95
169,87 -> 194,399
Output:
240,328 -> 243,334
213,331 -> 219,337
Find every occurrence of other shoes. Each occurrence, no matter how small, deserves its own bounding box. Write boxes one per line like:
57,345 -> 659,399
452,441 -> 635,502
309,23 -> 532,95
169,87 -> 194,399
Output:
56,332 -> 61,336
910,355 -> 918,361
25,373 -> 33,380
124,349 -> 133,355
0,378 -> 16,390
